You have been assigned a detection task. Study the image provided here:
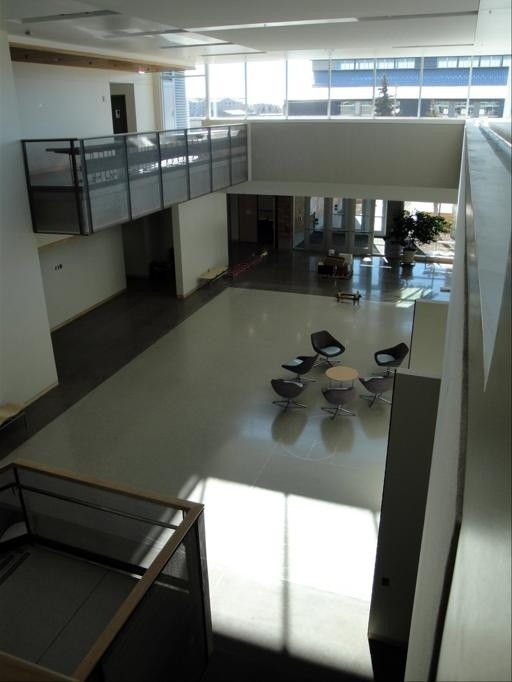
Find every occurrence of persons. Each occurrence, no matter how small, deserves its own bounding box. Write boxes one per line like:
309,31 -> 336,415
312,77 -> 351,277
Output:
353,291 -> 361,304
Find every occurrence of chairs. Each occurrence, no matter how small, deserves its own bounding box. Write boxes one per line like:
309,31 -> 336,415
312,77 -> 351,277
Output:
268,328 -> 409,422
315,252 -> 353,281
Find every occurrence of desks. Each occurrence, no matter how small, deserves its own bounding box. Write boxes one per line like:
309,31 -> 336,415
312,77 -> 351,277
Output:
198,264 -> 230,289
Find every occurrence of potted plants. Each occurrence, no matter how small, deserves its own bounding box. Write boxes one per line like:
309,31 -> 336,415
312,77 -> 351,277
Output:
381,208 -> 453,263
